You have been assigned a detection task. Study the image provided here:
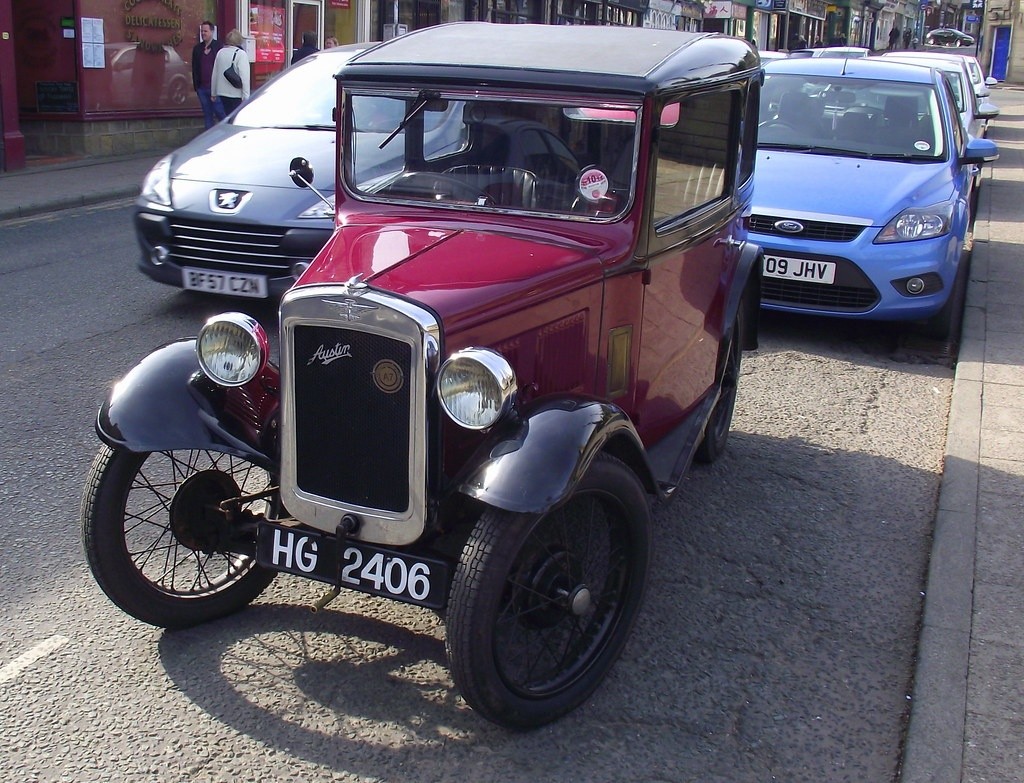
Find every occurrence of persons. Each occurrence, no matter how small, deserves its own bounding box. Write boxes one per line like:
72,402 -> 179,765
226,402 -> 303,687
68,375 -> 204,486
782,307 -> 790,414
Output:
787,34 -> 807,52
828,31 -> 847,47
887,27 -> 900,51
211,30 -> 250,116
904,28 -> 912,49
325,36 -> 339,49
81,26 -> 113,108
872,94 -> 929,152
751,39 -> 758,51
132,25 -> 166,107
291,30 -> 319,66
911,35 -> 919,49
783,91 -> 821,140
192,21 -> 227,131
813,36 -> 823,48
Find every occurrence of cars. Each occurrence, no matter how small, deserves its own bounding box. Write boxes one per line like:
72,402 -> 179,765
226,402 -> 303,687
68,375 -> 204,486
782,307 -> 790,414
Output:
746,42 -> 1002,165
926,27 -> 976,47
735,57 -> 1002,338
80,20 -> 767,731
133,40 -> 467,304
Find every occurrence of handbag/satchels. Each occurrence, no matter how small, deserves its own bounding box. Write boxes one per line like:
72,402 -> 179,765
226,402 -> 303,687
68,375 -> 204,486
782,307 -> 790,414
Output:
224,67 -> 243,89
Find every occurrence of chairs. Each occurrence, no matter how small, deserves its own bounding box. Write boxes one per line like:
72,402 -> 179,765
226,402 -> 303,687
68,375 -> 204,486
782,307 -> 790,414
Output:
597,132 -> 724,220
773,88 -> 933,149
434,165 -> 542,209
